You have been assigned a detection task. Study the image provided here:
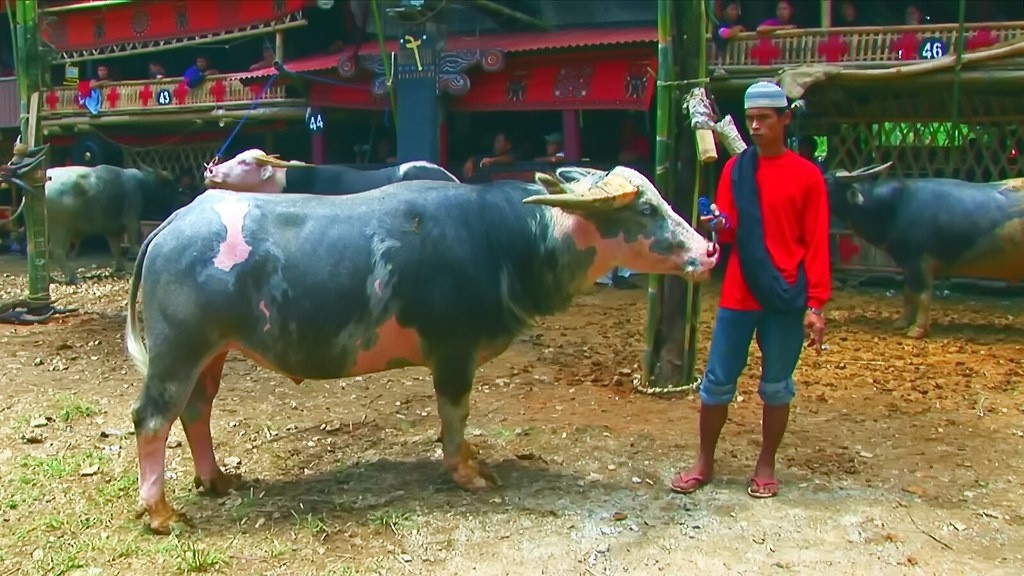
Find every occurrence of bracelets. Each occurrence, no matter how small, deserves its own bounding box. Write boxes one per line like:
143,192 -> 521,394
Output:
809,307 -> 822,316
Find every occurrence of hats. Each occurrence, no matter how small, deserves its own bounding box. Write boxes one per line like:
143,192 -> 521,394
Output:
744,81 -> 788,109
262,41 -> 270,48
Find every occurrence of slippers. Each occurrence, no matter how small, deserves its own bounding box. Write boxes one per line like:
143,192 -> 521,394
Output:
670,473 -> 716,492
747,477 -> 778,497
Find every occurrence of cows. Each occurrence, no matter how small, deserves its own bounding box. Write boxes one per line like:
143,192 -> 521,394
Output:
204,148 -> 461,197
822,161 -> 1024,339
124,164 -> 720,536
0,136 -> 206,284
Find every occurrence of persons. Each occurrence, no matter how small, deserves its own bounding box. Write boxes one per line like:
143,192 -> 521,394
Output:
797,132 -> 824,173
673,82 -> 831,498
64,154 -> 84,258
617,114 -> 652,163
147,55 -> 174,80
168,169 -> 197,212
247,36 -> 292,71
532,132 -> 567,164
0,59 -> 14,77
755,0 -> 796,35
463,132 -> 523,179
836,2 -> 859,28
716,0 -> 748,38
18,224 -> 28,256
372,136 -> 399,165
903,4 -> 930,26
90,62 -> 115,89
184,52 -> 221,89
